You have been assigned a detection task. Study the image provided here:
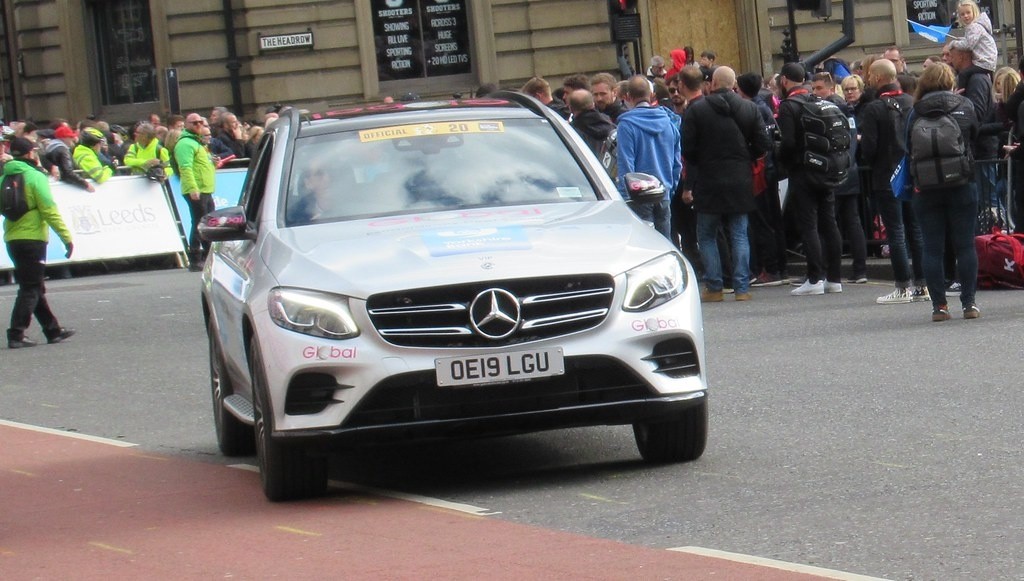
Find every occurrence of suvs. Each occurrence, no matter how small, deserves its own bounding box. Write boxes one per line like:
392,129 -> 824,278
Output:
199,86 -> 710,503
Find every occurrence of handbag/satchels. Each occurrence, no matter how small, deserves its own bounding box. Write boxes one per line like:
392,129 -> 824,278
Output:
974,226 -> 1024,290
752,161 -> 764,197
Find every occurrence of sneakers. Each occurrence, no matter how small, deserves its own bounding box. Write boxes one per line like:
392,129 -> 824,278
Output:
963,303 -> 980,318
877,287 -> 913,303
912,287 -> 931,301
932,306 -> 950,320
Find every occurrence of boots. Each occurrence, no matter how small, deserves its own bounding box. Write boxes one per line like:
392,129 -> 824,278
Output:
42,325 -> 76,344
7,328 -> 36,348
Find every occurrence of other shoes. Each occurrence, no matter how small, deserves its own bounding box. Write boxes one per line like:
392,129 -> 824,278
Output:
735,293 -> 750,300
701,288 -> 723,302
791,279 -> 824,296
824,280 -> 842,293
783,272 -> 790,284
847,275 -> 868,284
945,283 -> 961,296
189,261 -> 204,271
751,273 -> 782,287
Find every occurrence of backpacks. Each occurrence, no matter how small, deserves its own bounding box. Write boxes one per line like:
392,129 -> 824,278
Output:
909,97 -> 970,191
0,174 -> 28,222
787,97 -> 852,187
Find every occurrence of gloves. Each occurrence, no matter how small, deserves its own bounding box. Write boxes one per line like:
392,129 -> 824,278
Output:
66,242 -> 73,258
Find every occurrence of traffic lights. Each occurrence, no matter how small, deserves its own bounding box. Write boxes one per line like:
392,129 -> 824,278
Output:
612,0 -> 638,15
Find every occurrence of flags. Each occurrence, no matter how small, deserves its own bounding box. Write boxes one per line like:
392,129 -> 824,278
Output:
908,20 -> 952,45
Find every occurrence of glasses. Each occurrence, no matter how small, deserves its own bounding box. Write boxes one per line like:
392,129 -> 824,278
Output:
658,64 -> 665,69
668,87 -> 679,93
190,120 -> 203,126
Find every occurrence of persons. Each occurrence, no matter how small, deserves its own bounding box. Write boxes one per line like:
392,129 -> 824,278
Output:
0,103 -> 311,192
173,112 -> 216,271
288,160 -> 343,225
518,48 -> 1024,304
906,61 -> 980,322
945,0 -> 998,94
0,138 -> 76,348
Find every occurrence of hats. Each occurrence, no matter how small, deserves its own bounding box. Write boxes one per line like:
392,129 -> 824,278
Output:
10,138 -> 34,156
55,125 -> 77,138
36,128 -> 54,139
737,73 -> 762,98
80,127 -> 104,146
779,62 -> 805,82
